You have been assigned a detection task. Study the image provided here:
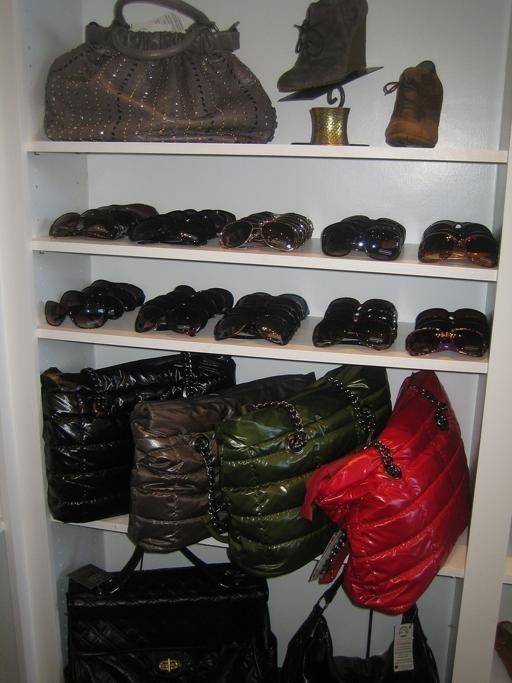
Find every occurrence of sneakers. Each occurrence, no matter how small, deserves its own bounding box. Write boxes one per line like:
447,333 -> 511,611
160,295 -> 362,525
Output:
384,60 -> 443,149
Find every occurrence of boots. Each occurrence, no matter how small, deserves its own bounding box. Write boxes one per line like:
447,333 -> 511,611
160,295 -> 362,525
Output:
278,0 -> 366,92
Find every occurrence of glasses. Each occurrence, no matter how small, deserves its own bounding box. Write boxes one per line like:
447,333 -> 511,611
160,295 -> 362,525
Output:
419,219 -> 497,268
135,284 -> 233,337
404,308 -> 489,359
321,215 -> 405,261
312,297 -> 397,351
45,279 -> 146,328
223,211 -> 314,251
50,200 -> 158,241
213,292 -> 310,346
127,210 -> 238,247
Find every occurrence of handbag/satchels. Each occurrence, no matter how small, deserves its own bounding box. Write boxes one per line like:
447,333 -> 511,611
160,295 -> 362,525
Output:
212,366 -> 393,578
62,548 -> 278,683
44,0 -> 278,142
125,353 -> 317,556
301,370 -> 468,616
40,352 -> 238,525
282,614 -> 440,682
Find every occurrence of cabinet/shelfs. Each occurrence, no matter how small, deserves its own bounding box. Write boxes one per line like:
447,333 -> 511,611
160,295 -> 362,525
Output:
25,138 -> 508,581
